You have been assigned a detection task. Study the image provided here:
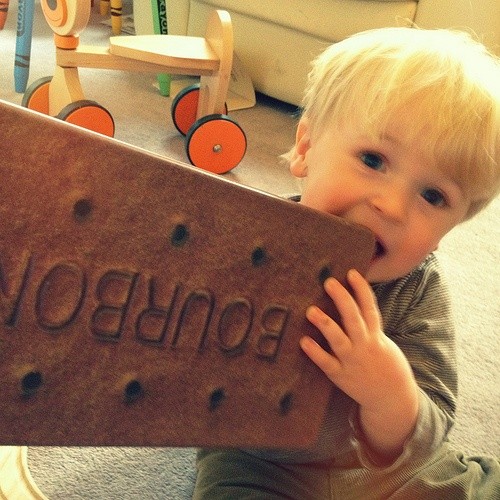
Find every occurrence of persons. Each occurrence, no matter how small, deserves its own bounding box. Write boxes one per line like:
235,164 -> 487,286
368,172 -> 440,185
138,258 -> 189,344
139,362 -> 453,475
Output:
187,17 -> 500,499
